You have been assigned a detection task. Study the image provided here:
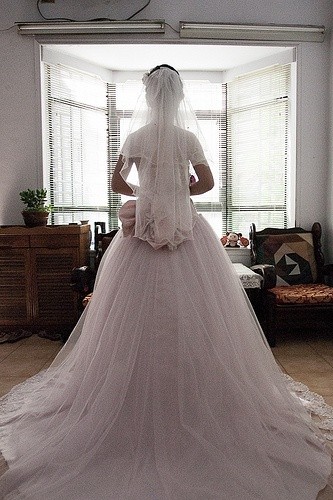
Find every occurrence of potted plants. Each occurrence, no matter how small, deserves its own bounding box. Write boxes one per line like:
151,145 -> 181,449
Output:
18,187 -> 50,227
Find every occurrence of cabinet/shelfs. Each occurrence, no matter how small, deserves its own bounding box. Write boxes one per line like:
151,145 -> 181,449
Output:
0,224 -> 93,330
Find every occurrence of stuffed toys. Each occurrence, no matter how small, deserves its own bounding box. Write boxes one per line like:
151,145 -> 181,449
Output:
226,232 -> 242,248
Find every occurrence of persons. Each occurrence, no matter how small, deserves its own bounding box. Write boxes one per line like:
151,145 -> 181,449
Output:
0,65 -> 333,500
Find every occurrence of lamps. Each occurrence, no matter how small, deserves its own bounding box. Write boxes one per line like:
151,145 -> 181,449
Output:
179,21 -> 327,42
13,19 -> 166,34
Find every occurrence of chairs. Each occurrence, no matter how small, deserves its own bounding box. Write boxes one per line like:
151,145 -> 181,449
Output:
248,222 -> 333,347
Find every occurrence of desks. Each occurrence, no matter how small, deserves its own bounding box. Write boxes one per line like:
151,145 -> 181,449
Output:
232,262 -> 263,309
